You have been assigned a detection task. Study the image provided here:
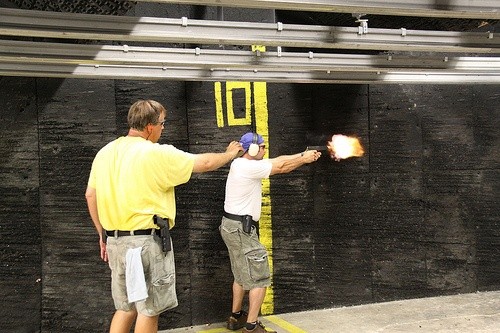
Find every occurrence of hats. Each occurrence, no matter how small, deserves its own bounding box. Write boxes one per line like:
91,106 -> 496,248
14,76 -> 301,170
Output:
237,132 -> 264,157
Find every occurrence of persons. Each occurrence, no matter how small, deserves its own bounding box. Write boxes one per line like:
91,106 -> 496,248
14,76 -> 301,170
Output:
219,133 -> 322,333
85,100 -> 245,333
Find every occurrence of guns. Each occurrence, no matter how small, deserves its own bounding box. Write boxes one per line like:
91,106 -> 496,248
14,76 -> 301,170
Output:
307,146 -> 329,150
153,215 -> 171,252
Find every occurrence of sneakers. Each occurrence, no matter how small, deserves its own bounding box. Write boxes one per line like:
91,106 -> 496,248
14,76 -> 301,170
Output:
227,310 -> 248,330
242,321 -> 277,333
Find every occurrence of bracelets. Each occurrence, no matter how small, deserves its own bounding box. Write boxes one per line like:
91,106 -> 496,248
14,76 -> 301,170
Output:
301,153 -> 304,157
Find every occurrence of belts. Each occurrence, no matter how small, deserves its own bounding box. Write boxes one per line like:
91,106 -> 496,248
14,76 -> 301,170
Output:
106,228 -> 160,237
223,212 -> 257,227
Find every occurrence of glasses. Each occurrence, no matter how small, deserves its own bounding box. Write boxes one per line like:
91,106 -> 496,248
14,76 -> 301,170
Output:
158,120 -> 167,126
259,145 -> 265,148
